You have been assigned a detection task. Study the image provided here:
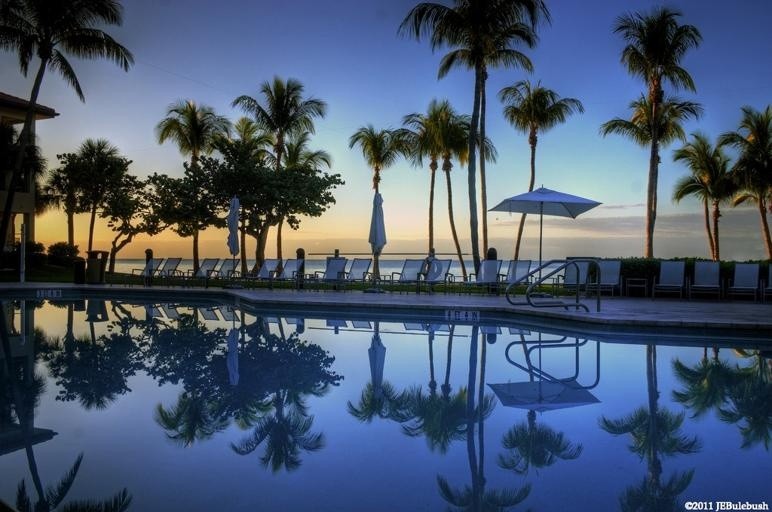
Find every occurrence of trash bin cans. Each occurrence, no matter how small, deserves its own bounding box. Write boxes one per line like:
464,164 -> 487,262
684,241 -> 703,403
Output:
86,250 -> 109,282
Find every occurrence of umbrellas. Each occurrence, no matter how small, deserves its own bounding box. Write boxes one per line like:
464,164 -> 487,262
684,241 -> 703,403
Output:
225,194 -> 241,279
366,321 -> 388,404
368,192 -> 387,288
486,329 -> 601,417
487,182 -> 605,292
225,307 -> 241,389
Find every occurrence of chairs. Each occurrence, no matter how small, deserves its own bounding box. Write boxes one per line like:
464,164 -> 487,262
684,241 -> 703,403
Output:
651,260 -> 772,304
121,255 -> 624,301
